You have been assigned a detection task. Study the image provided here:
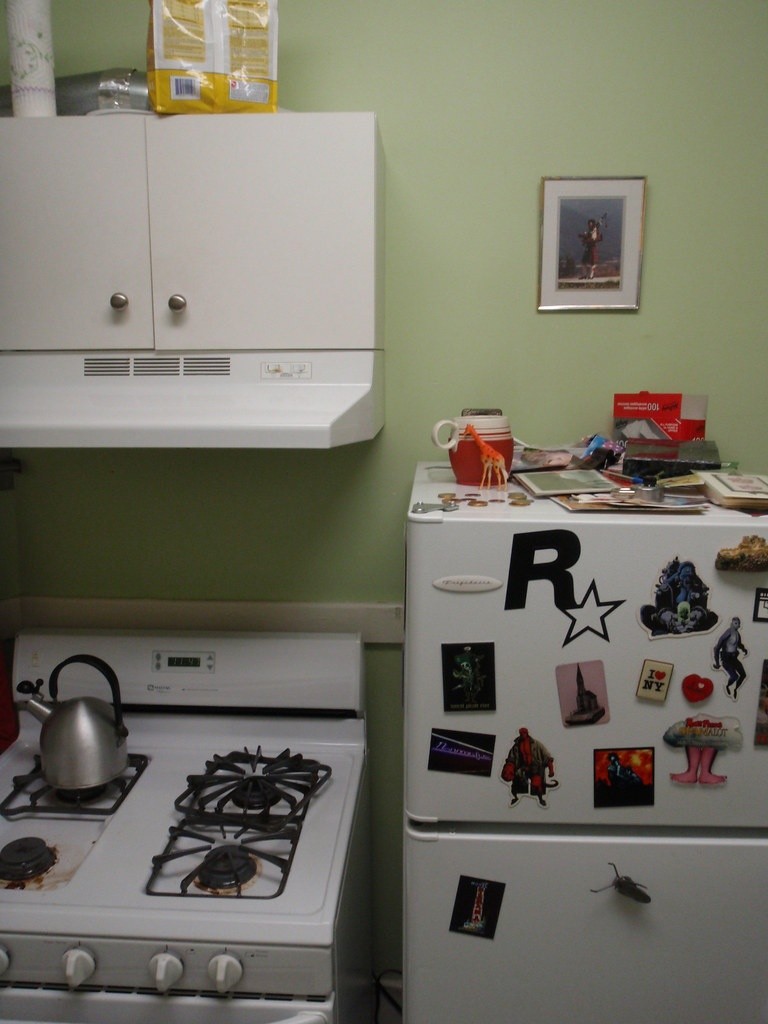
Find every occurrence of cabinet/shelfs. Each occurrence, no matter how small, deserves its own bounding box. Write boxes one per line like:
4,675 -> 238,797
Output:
2,110 -> 385,451
396,461 -> 768,1024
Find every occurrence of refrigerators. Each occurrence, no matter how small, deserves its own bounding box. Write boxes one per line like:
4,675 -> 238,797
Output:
391,453 -> 766,1024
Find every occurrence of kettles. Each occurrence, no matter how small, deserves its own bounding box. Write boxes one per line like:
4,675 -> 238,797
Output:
11,651 -> 132,797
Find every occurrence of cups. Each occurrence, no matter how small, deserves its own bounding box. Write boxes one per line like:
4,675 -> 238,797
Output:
431,414 -> 512,488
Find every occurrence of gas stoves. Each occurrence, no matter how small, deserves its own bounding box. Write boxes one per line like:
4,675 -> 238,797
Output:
1,621 -> 373,1007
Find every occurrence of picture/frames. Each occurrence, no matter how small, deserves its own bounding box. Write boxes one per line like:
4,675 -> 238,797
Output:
537,176 -> 646,312
